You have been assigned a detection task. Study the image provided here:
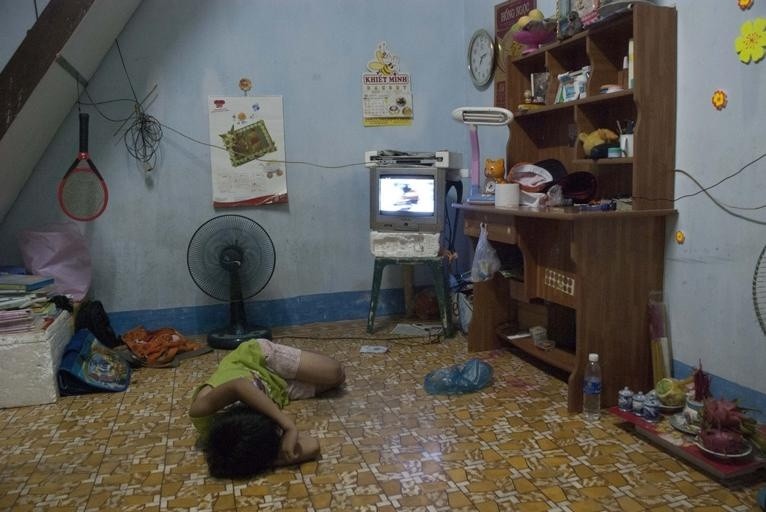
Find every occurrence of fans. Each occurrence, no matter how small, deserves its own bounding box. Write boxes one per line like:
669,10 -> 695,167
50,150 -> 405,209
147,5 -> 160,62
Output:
751,241 -> 766,334
184,211 -> 278,352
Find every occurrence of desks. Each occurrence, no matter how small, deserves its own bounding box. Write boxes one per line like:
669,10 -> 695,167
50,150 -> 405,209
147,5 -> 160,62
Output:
447,197 -> 680,420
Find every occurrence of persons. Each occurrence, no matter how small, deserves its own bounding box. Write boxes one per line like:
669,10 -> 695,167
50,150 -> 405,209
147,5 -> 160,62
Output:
187,337 -> 346,480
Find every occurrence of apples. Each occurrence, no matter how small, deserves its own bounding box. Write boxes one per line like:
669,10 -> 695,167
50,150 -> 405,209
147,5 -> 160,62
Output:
517,16 -> 529,28
528,8 -> 543,21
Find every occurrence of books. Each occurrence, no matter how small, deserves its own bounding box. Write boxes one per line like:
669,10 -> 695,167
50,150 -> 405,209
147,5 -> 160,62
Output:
0,271 -> 57,338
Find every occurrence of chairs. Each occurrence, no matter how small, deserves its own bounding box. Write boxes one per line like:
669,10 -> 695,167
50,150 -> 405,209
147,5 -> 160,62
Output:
363,180 -> 465,341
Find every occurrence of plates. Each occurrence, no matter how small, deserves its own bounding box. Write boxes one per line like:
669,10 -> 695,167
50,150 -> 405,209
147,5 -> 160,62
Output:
649,388 -> 684,412
695,436 -> 753,463
671,412 -> 697,436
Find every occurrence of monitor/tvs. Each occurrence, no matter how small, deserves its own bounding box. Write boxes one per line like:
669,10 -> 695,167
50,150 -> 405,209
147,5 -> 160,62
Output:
369,165 -> 446,232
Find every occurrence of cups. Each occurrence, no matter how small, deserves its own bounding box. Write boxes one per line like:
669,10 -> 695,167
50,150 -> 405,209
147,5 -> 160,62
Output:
657,376 -> 690,405
685,398 -> 707,432
620,134 -> 635,158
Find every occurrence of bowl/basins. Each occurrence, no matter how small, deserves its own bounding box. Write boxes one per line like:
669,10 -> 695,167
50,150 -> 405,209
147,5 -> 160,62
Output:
597,1 -> 657,18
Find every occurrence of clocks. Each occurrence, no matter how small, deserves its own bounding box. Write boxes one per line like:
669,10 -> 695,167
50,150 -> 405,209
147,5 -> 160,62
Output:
465,27 -> 497,89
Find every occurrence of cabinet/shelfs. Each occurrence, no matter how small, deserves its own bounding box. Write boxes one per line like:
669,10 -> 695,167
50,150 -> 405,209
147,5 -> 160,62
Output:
501,0 -> 679,212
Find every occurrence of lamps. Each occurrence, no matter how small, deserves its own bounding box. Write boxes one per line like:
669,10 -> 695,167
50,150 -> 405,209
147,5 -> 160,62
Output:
450,102 -> 514,205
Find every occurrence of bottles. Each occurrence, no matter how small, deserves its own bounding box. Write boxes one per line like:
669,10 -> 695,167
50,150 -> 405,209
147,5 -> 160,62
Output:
618,386 -> 659,422
582,353 -> 602,419
